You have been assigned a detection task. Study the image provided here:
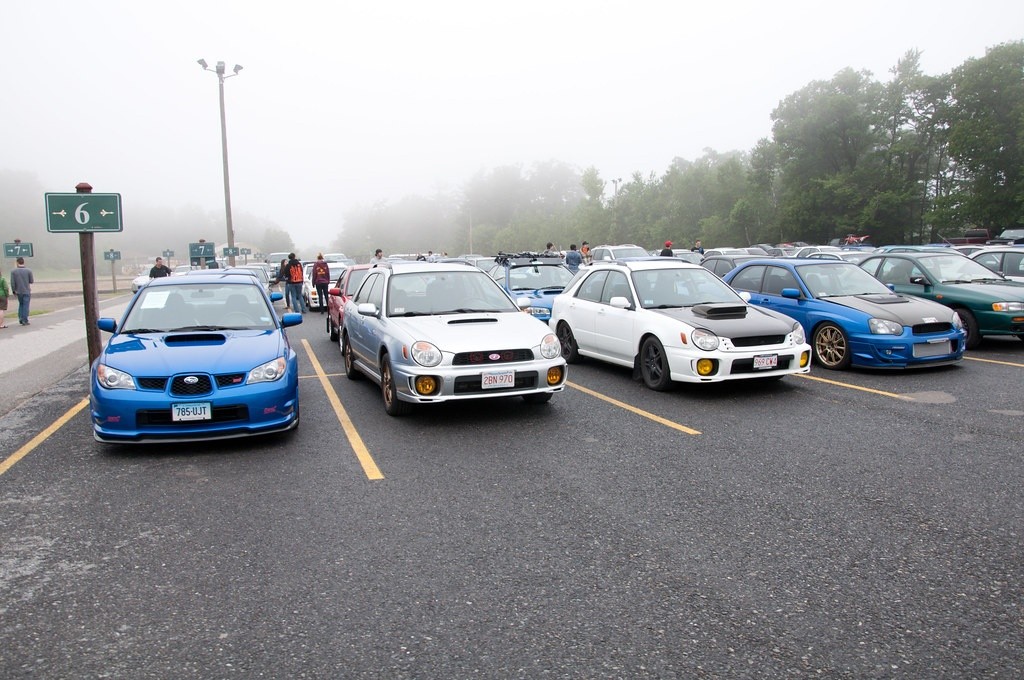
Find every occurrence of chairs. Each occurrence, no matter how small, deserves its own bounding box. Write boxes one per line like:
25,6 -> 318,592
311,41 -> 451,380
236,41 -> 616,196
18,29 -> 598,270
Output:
764,275 -> 822,296
165,293 -> 187,312
226,293 -> 248,313
1006,254 -> 1020,275
888,265 -> 909,285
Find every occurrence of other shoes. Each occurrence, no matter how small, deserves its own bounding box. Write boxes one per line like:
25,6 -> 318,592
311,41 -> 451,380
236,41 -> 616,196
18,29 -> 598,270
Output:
284,306 -> 290,309
20,321 -> 30,325
0,325 -> 8,328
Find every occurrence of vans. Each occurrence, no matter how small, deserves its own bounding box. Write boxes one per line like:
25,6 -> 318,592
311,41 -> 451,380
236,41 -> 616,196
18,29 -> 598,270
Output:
265,252 -> 291,277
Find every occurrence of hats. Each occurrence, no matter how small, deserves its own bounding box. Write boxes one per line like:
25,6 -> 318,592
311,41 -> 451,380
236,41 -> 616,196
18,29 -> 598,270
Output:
665,241 -> 673,246
582,241 -> 589,245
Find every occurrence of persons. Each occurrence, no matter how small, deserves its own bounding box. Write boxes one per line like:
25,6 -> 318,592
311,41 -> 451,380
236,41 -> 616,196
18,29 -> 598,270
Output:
543,240 -> 592,274
370,249 -> 388,265
149,257 -> 172,281
11,257 -> 34,325
415,251 -> 449,263
659,241 -> 674,257
0,269 -> 10,328
691,240 -> 704,255
272,253 -> 330,314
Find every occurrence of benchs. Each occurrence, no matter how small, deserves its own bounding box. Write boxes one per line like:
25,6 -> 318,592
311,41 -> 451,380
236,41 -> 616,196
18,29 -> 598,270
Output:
734,278 -> 763,292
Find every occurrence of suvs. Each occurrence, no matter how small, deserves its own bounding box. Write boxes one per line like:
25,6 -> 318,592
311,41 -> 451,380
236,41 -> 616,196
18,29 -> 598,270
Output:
589,244 -> 648,259
548,257 -> 812,393
341,259 -> 568,418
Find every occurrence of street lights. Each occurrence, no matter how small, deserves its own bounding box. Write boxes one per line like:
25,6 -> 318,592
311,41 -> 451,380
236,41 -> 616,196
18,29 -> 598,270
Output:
196,57 -> 243,265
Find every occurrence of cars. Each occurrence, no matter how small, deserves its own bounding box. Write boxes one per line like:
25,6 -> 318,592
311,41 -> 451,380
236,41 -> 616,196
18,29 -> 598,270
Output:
88,276 -> 304,444
938,249 -> 1024,283
131,269 -> 149,294
843,251 -> 1024,353
164,263 -> 274,308
299,259 -> 381,356
391,229 -> 1024,323
678,259 -> 967,370
